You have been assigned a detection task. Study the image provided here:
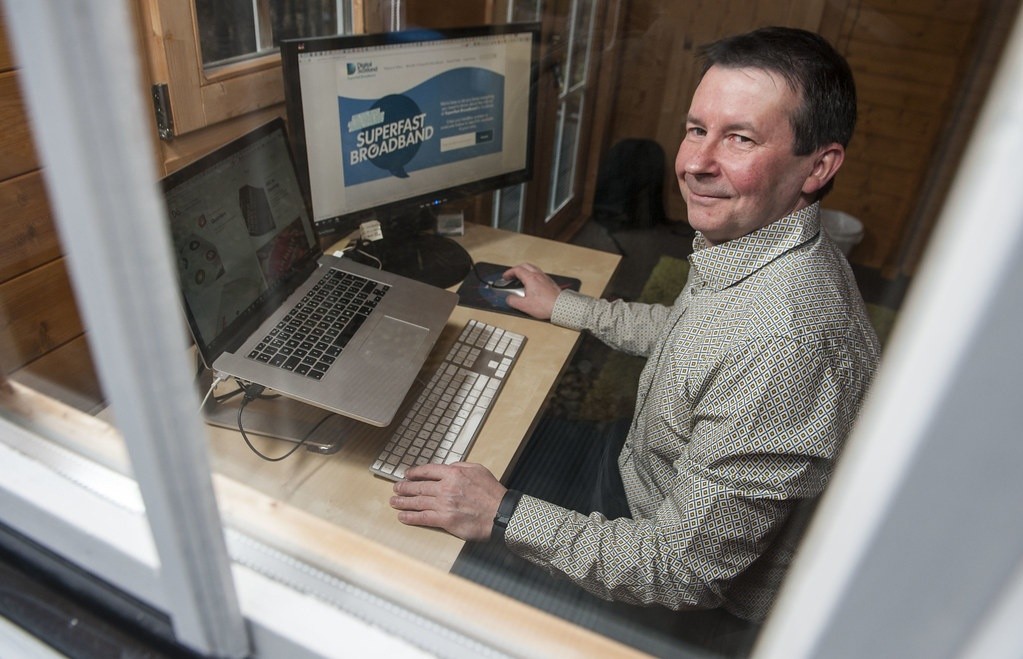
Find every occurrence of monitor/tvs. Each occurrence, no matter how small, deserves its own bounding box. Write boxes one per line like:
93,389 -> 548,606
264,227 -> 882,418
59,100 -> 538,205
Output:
278,22 -> 543,289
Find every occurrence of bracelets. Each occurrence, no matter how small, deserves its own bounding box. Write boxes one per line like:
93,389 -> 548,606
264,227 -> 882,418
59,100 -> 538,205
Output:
491,489 -> 522,545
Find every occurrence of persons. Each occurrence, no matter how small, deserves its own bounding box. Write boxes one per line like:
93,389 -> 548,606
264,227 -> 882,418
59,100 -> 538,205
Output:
390,26 -> 882,659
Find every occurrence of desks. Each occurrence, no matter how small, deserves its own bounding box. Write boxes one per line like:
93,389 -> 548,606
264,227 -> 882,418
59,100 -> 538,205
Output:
103,219 -> 622,562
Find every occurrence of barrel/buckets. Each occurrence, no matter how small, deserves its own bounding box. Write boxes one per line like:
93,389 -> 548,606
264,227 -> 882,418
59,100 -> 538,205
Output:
821,208 -> 864,262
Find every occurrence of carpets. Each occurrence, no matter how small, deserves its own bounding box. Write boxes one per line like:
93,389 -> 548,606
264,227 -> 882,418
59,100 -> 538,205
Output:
576,256 -> 899,419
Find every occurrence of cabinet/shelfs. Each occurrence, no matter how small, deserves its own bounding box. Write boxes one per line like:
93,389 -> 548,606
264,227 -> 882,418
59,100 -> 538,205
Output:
406,0 -> 624,240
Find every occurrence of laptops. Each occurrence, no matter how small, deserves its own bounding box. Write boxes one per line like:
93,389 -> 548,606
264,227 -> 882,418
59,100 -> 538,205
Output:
159,116 -> 461,426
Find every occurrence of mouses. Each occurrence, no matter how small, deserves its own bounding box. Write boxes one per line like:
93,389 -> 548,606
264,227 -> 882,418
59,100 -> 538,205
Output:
492,276 -> 528,301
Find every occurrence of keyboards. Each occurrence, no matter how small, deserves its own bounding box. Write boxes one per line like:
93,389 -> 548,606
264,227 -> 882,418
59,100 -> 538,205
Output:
369,319 -> 527,482
